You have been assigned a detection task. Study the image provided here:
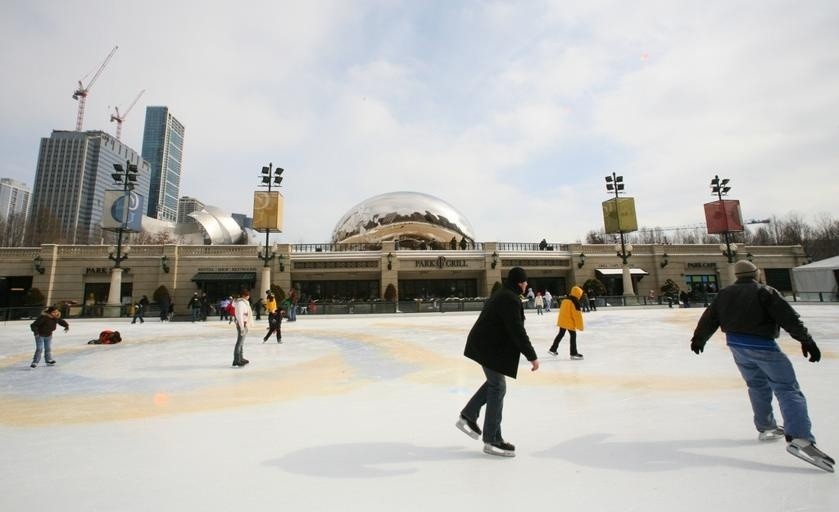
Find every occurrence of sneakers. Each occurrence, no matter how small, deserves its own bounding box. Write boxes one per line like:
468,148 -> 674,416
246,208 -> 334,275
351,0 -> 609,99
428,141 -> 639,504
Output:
490,441 -> 515,452
459,412 -> 482,436
233,359 -> 249,366
757,426 -> 785,437
550,349 -> 558,355
785,433 -> 835,466
30,362 -> 39,368
570,353 -> 583,357
45,360 -> 56,364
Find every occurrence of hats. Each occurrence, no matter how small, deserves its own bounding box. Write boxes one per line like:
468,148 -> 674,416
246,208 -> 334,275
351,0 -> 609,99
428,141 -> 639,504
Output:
509,267 -> 527,283
733,260 -> 761,279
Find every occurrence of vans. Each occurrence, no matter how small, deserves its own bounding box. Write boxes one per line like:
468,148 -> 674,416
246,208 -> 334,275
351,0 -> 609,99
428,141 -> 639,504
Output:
0,287 -> 45,320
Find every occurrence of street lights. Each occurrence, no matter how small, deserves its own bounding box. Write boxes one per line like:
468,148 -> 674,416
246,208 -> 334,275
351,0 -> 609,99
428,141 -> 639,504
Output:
252,162 -> 285,267
101,160 -> 141,269
602,172 -> 638,265
703,175 -> 744,264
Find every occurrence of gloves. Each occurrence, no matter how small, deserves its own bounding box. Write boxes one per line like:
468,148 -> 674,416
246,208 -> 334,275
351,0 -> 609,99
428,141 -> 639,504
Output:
801,335 -> 821,363
690,336 -> 706,354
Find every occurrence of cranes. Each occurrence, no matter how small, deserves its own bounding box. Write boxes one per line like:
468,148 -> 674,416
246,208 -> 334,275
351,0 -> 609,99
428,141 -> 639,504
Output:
600,219 -> 770,243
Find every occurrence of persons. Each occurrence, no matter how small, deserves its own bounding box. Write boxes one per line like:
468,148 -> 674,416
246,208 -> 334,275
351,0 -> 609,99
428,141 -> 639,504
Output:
547,286 -> 584,358
455,266 -> 539,457
94,296 -> 106,317
667,289 -> 674,307
534,290 -> 544,315
587,286 -> 597,311
200,292 -> 210,321
580,287 -> 590,312
187,291 -> 204,322
648,288 -> 657,305
690,260 -> 836,472
85,292 -> 95,307
542,288 -> 553,312
157,292 -> 170,323
28,306 -> 69,368
169,301 -> 175,321
519,293 -> 528,301
703,283 -> 714,292
526,288 -> 534,298
680,290 -> 690,308
130,301 -> 144,324
139,294 -> 149,311
219,285 -> 297,368
87,330 -> 121,344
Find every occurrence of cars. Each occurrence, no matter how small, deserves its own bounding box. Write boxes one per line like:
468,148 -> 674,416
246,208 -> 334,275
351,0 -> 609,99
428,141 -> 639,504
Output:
399,238 -> 421,250
